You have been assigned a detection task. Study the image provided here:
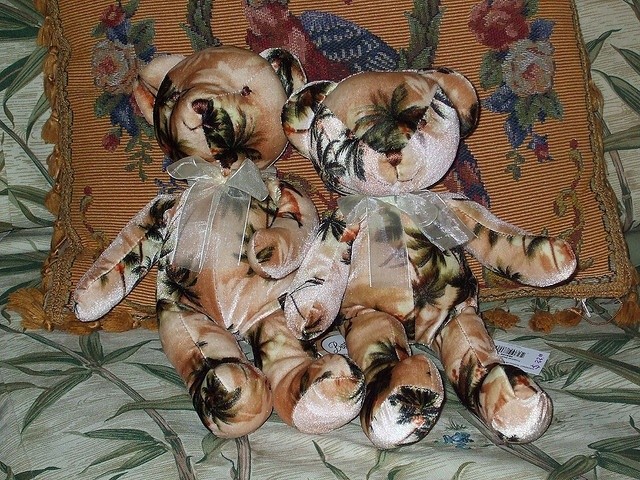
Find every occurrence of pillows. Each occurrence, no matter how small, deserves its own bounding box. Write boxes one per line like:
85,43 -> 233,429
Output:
8,0 -> 640,335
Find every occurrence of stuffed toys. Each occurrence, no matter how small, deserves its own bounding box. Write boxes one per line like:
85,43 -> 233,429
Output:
279,64 -> 580,450
70,47 -> 367,439
